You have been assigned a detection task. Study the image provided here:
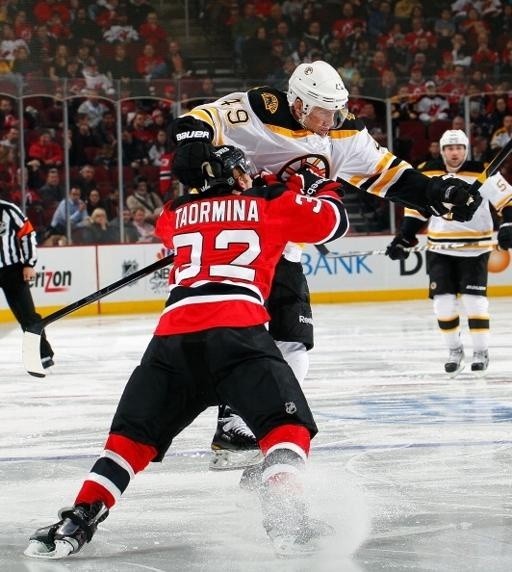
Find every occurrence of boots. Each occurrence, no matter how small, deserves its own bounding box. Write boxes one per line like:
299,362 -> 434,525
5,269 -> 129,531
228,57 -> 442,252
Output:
259,494 -> 337,539
29,502 -> 110,554
209,403 -> 261,451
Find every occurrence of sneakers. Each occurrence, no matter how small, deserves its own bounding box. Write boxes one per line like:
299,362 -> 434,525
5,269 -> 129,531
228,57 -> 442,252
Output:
42,357 -> 54,369
471,349 -> 490,371
444,345 -> 465,373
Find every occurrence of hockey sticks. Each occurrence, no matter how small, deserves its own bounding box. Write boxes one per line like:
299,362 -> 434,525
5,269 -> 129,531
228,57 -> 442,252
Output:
22,252 -> 174,378
314,240 -> 499,258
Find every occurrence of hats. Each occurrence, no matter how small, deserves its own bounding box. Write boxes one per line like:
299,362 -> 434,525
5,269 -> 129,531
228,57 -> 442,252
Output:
425,80 -> 435,88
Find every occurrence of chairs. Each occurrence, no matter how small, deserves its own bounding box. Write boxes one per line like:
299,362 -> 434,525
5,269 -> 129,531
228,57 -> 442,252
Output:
0,40 -> 162,223
396,118 -> 451,171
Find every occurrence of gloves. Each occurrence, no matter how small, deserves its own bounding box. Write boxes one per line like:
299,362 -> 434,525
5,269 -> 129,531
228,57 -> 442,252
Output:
171,140 -> 222,187
427,175 -> 483,223
286,167 -> 346,198
389,231 -> 418,261
497,222 -> 512,251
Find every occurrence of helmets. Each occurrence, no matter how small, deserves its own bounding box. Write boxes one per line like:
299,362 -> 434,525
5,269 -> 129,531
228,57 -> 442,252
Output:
286,61 -> 350,130
197,145 -> 257,194
438,128 -> 469,172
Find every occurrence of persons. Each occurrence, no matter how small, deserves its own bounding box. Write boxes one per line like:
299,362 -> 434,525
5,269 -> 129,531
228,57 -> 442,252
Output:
151,58 -> 484,464
0,199 -> 56,372
26,143 -> 350,555
382,127 -> 512,374
1,1 -> 512,249
